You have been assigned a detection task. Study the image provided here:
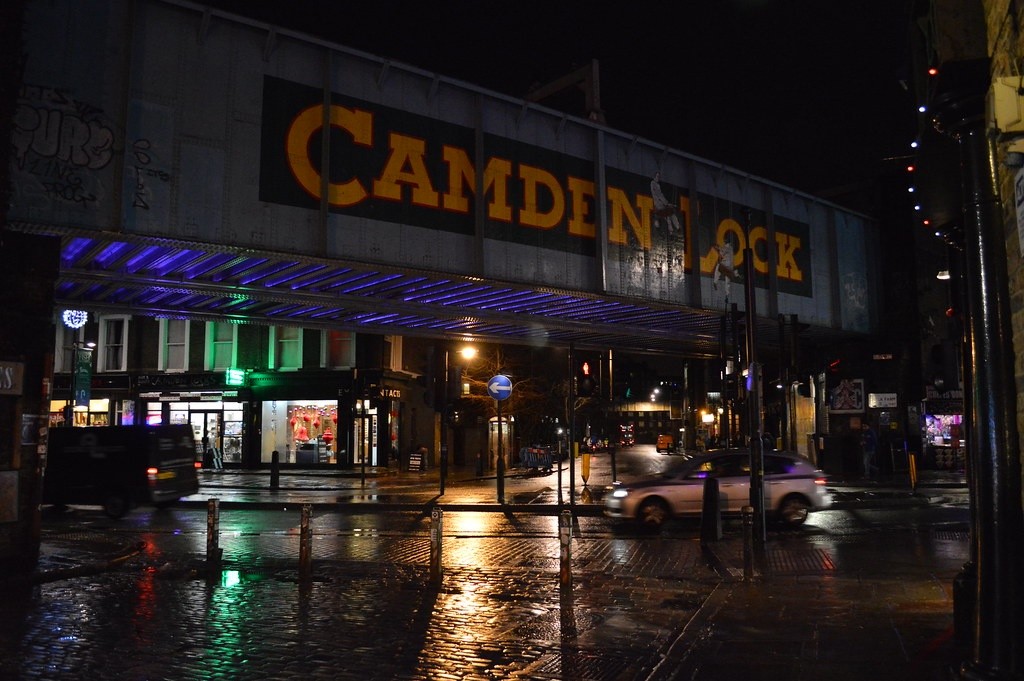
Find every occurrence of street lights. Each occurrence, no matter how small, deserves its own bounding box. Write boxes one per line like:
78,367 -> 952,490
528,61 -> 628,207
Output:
68,342 -> 96,426
440,343 -> 480,494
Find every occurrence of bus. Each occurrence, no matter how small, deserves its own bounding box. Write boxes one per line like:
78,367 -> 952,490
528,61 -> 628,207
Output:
623,430 -> 634,447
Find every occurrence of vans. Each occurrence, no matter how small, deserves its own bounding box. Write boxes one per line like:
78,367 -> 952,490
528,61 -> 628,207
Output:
656,435 -> 674,453
40,424 -> 201,518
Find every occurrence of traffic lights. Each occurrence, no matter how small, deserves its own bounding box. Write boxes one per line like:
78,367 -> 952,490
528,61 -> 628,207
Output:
794,364 -> 816,400
581,360 -> 596,395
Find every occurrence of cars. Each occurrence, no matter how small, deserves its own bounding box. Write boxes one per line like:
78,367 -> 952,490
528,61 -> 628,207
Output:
602,450 -> 831,528
